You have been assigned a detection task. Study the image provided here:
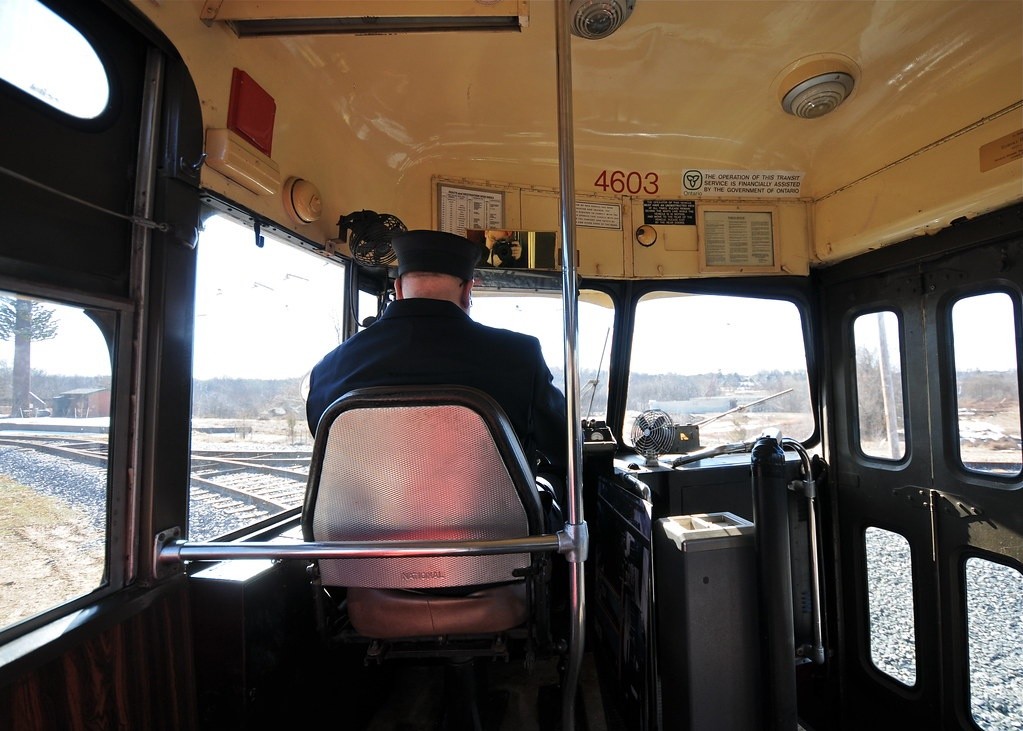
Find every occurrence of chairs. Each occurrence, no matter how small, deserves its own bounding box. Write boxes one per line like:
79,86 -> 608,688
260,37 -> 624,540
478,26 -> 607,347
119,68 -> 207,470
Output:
301,381 -> 570,731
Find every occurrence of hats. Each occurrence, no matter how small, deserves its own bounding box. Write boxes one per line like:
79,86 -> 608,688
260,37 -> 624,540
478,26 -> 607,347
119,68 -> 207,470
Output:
391,230 -> 482,281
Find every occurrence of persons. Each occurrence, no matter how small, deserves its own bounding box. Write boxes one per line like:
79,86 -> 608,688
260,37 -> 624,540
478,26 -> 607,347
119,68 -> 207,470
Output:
305,223 -> 578,685
481,230 -> 522,268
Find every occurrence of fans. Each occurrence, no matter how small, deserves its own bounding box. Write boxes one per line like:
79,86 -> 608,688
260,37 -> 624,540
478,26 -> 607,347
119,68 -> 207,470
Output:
631,408 -> 675,467
